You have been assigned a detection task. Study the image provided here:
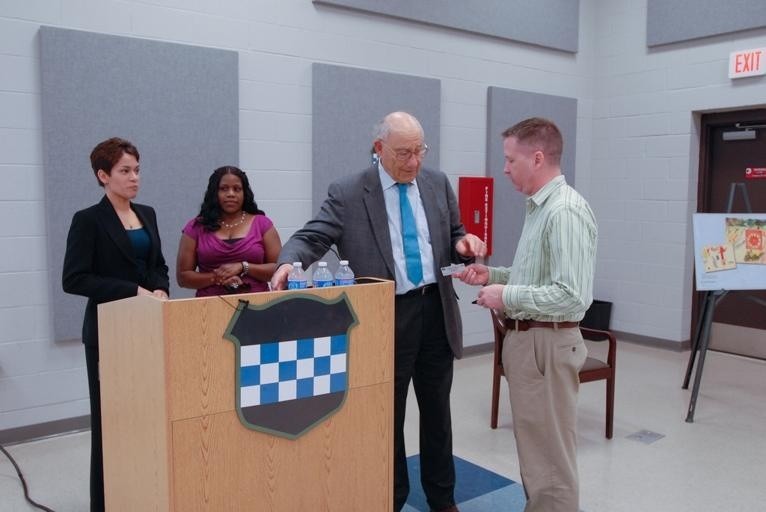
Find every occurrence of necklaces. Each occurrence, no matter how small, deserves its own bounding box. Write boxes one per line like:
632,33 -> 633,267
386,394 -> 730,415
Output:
219,209 -> 246,228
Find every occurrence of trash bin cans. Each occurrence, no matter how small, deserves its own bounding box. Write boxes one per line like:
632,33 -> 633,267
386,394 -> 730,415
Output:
579,299 -> 613,341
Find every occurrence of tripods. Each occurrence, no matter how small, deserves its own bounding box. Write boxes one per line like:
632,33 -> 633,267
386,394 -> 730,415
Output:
681,182 -> 766,423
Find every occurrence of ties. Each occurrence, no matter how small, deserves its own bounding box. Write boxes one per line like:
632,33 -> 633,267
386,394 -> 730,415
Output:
397,184 -> 424,285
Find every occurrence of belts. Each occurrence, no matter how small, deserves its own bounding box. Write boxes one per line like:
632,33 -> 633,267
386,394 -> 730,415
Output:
410,284 -> 436,297
503,317 -> 579,330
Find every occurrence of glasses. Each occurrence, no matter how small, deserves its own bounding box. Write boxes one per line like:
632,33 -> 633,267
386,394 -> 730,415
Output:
381,142 -> 428,161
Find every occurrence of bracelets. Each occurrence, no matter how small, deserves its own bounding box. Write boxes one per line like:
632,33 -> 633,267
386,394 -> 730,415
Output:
240,260 -> 249,277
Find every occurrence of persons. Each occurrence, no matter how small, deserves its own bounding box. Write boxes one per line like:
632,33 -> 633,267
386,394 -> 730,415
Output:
272,111 -> 488,510
176,165 -> 283,297
62,136 -> 168,511
451,116 -> 599,512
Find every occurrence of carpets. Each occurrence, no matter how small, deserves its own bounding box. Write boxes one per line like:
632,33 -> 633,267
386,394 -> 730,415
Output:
395,450 -> 527,512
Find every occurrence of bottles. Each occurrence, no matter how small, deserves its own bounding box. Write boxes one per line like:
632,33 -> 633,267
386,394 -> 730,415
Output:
312,261 -> 334,288
287,260 -> 309,289
335,259 -> 356,286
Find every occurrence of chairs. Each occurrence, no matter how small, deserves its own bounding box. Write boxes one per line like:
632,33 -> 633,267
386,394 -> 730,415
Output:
488,300 -> 618,441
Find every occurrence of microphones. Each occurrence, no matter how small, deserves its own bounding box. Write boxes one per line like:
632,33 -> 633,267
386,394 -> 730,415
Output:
308,234 -> 341,262
